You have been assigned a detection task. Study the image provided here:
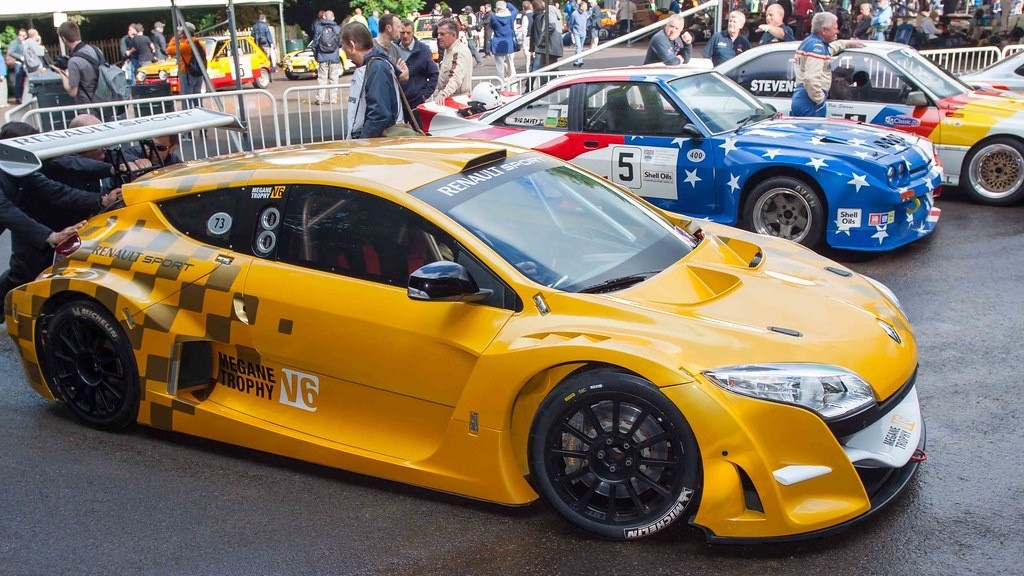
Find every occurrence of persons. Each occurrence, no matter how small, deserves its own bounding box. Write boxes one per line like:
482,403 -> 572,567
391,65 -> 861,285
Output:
643,13 -> 693,66
700,10 -> 752,67
788,11 -> 867,117
340,22 -> 405,139
754,3 -> 795,45
827,64 -> 853,100
0,0 -> 1024,325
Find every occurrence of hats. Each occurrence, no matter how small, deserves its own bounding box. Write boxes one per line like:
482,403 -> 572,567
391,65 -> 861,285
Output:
180,21 -> 196,32
154,21 -> 166,27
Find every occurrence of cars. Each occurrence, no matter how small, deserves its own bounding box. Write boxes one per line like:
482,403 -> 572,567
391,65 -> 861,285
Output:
560,8 -> 618,34
136,35 -> 273,93
711,40 -> 1024,205
957,48 -> 1024,91
417,68 -> 942,251
414,16 -> 480,61
476,15 -> 522,51
687,11 -> 764,45
281,40 -> 357,80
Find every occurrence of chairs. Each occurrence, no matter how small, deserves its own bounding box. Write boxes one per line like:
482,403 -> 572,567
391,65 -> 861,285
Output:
406,225 -> 459,282
638,84 -> 665,120
325,218 -> 387,276
607,88 -> 643,131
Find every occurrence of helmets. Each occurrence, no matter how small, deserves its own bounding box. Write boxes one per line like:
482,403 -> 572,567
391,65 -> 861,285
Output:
470,82 -> 503,112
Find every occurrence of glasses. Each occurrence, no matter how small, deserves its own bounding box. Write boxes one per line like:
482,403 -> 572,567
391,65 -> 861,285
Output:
156,144 -> 173,151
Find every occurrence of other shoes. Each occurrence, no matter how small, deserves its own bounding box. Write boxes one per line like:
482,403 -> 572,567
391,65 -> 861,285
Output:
16,99 -> 21,104
315,100 -> 323,105
1,104 -> 10,107
574,63 -> 584,68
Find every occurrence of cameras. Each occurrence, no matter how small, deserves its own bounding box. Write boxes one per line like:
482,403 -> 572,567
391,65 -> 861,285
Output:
51,56 -> 69,72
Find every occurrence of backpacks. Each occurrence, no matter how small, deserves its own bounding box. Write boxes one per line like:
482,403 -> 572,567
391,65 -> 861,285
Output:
181,40 -> 208,85
73,43 -> 131,117
317,24 -> 339,53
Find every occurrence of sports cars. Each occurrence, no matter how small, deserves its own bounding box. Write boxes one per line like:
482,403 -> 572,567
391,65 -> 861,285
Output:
0,107 -> 929,542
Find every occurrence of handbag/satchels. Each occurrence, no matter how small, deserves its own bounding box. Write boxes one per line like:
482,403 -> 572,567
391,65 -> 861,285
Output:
514,43 -> 520,52
351,124 -> 423,138
24,40 -> 40,68
259,36 -> 268,44
